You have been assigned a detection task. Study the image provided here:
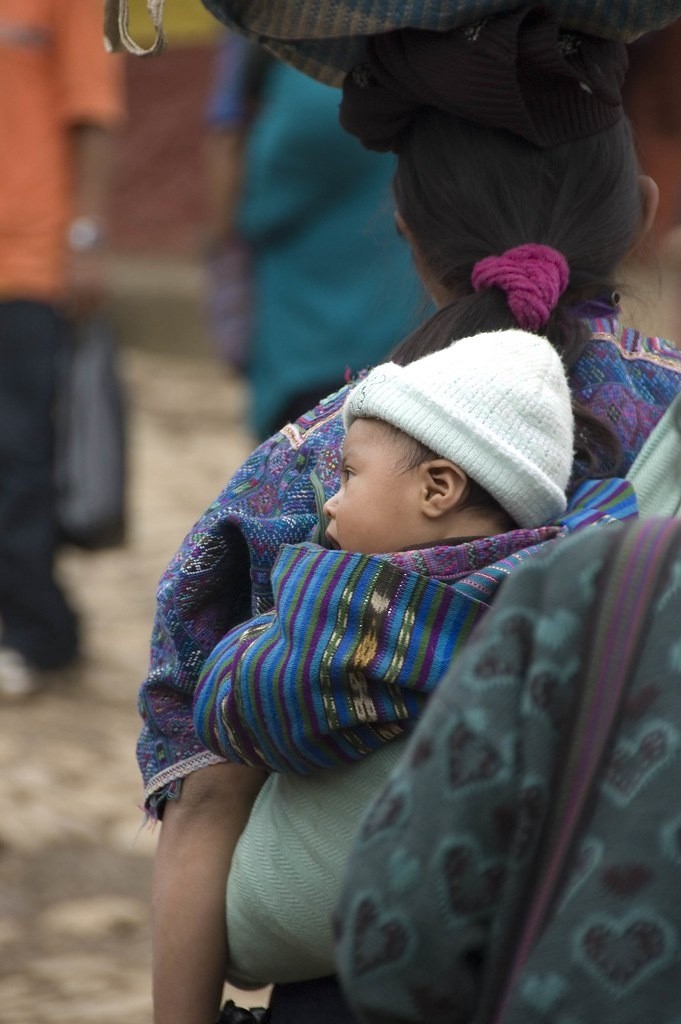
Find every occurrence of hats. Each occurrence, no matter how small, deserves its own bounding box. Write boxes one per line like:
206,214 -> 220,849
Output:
342,326 -> 574,529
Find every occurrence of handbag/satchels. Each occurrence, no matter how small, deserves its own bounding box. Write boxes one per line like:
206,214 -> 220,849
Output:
59,224 -> 126,552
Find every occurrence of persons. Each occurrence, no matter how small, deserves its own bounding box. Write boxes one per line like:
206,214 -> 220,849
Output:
0,0 -> 130,707
139,0 -> 681,1024
